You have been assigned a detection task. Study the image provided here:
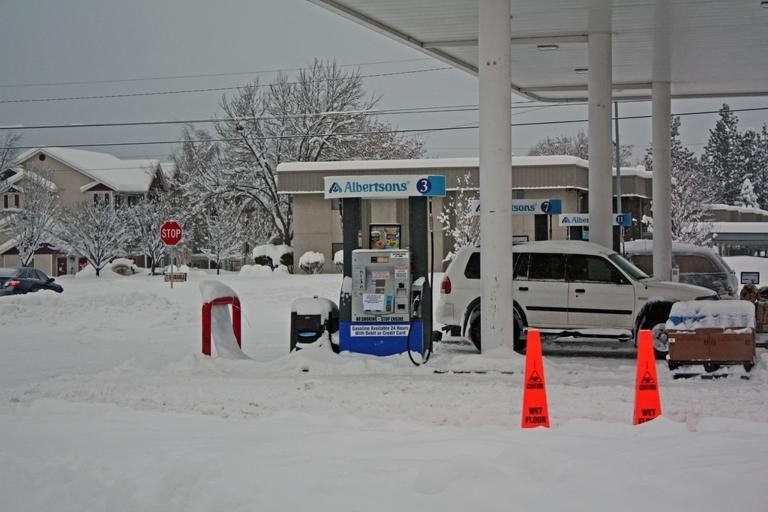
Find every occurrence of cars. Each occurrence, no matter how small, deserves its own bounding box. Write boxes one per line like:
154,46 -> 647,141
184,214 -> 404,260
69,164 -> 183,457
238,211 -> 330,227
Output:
113,258 -> 137,278
0,265 -> 68,299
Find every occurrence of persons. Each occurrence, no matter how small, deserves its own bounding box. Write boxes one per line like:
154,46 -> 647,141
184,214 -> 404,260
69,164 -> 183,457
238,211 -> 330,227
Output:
739,281 -> 759,305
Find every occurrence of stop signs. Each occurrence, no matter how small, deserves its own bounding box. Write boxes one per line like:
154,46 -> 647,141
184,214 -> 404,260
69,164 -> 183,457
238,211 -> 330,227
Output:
160,220 -> 184,248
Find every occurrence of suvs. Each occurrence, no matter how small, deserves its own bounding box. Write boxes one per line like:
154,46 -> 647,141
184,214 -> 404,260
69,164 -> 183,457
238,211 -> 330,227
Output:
433,241 -> 720,362
611,235 -> 739,302
